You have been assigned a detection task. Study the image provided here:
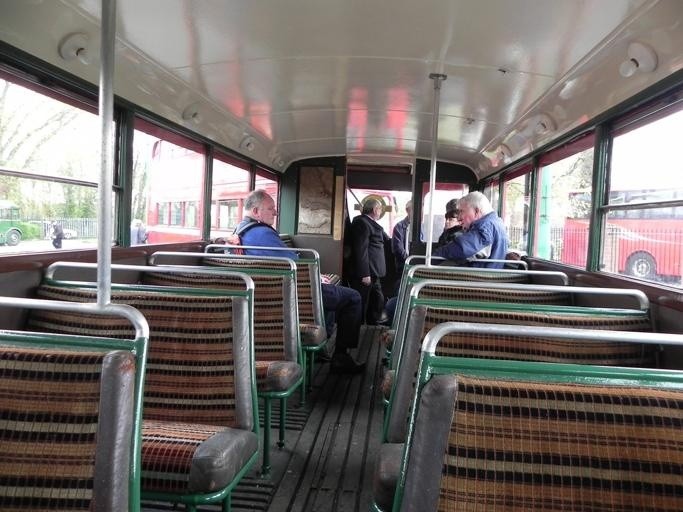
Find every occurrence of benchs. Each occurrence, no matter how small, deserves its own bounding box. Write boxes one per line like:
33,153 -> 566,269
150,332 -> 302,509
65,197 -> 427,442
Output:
378,255 -> 683,512
1,295 -> 150,512
137,249 -> 306,453
23,260 -> 260,512
204,232 -> 340,392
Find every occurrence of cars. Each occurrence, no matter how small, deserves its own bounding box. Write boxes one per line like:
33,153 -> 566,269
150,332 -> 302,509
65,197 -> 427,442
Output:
28,221 -> 76,240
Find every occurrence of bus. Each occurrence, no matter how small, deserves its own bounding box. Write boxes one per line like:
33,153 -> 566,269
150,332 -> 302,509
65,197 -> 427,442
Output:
561,189 -> 683,279
146,139 -> 399,244
0,201 -> 23,246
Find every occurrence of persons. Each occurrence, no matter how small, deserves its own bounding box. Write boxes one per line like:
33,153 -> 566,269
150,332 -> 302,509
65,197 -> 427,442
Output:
51,220 -> 63,248
391,201 -> 424,274
437,210 -> 464,246
349,198 -> 387,324
376,191 -> 507,329
445,198 -> 459,212
235,190 -> 368,375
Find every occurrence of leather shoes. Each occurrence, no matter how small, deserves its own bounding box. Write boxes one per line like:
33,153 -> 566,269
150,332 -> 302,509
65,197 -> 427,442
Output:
376,318 -> 393,326
330,353 -> 370,371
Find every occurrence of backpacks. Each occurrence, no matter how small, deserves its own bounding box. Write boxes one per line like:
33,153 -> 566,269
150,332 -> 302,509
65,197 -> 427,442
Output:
209,223 -> 277,256
